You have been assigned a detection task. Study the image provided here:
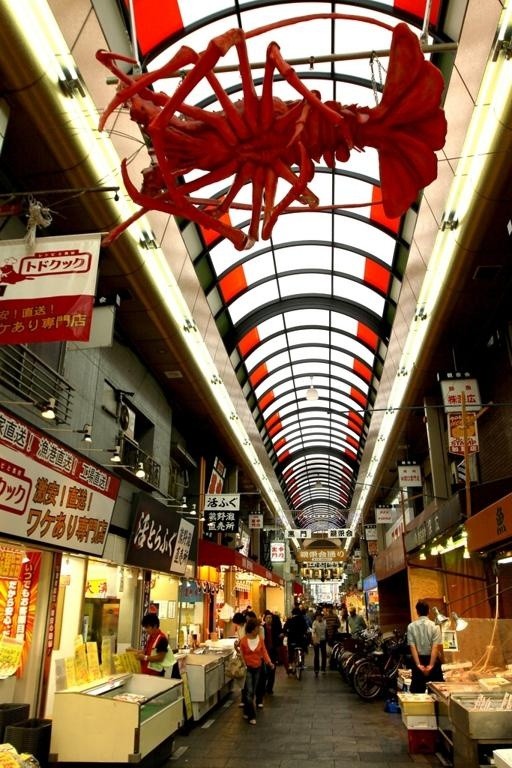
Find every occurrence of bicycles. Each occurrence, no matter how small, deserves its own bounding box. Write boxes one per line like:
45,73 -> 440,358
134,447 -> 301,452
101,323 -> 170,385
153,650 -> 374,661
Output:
284,633 -> 306,682
329,623 -> 429,701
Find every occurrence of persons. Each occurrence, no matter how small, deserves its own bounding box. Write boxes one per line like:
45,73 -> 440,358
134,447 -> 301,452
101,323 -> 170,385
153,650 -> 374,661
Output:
406,600 -> 445,693
126,614 -> 168,676
231,599 -> 366,725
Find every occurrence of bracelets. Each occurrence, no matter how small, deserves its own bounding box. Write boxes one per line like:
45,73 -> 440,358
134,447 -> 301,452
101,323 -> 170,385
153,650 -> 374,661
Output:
144,655 -> 148,661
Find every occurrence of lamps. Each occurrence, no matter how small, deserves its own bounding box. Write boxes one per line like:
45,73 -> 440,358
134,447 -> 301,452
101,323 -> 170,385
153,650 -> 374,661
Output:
432,576 -> 504,625
0,398 -> 144,478
452,586 -> 512,631
154,496 -> 205,521
306,376 -> 318,400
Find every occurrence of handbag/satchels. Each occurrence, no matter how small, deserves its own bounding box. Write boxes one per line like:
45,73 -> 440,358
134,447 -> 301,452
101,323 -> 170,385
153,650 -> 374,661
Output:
150,634 -> 177,668
224,652 -> 248,680
